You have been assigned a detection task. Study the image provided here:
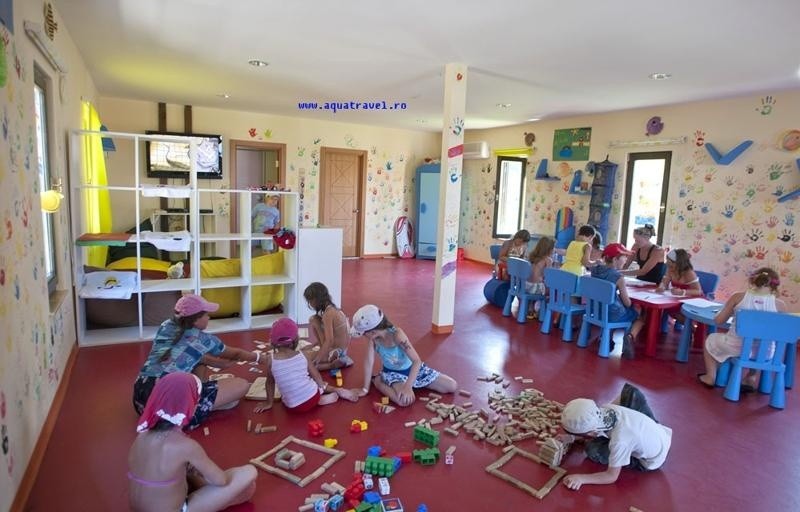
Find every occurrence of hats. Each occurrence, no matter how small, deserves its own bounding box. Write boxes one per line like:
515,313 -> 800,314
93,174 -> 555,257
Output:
269,317 -> 300,348
349,303 -> 385,339
601,243 -> 636,259
560,397 -> 618,435
173,293 -> 221,320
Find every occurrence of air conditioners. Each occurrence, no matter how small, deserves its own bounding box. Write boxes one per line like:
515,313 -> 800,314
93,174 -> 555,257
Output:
463,142 -> 489,159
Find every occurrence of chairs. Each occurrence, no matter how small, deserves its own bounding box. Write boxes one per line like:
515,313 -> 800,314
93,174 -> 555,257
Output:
577,276 -> 631,358
540,268 -> 586,342
716,309 -> 800,410
664,271 -> 719,331
490,244 -> 502,279
503,257 -> 546,324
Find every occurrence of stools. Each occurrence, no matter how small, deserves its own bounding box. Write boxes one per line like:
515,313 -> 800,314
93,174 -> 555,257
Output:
484,279 -> 509,308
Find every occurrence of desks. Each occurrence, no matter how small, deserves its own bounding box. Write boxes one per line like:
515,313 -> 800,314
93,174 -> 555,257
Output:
676,302 -> 796,390
616,284 -> 706,356
298,228 -> 342,325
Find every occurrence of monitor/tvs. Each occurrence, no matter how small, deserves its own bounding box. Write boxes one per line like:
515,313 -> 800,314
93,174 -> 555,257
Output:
146,130 -> 222,179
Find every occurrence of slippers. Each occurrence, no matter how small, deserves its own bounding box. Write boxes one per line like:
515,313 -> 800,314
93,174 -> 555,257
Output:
696,373 -> 716,389
741,383 -> 756,395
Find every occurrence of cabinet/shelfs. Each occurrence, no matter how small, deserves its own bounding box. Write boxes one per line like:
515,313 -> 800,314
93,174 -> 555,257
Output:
416,164 -> 440,260
68,130 -> 298,347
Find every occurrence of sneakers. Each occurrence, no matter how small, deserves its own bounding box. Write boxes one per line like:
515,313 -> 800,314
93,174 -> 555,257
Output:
526,309 -> 615,348
622,333 -> 636,361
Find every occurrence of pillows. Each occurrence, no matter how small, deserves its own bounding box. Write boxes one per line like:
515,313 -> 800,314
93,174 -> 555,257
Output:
82,218 -> 286,326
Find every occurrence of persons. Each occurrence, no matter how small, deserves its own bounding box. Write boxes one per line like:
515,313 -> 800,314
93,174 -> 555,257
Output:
496,223 -> 702,359
699,267 -> 790,387
128,371 -> 257,511
250,193 -> 280,255
132,293 -> 269,430
350,304 -> 457,407
299,282 -> 351,371
559,381 -> 673,490
202,338 -> 565,511
253,319 -> 359,413
